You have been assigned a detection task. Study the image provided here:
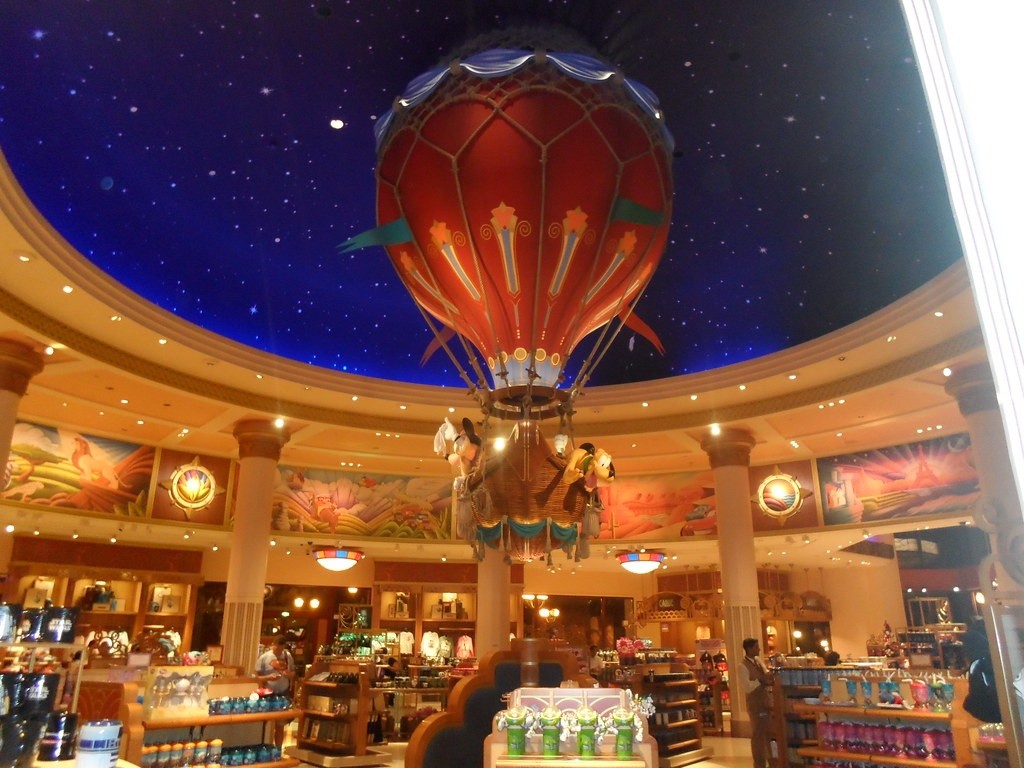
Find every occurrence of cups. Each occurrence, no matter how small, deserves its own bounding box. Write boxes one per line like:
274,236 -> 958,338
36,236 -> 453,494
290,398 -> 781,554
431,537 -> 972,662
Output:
613,705 -> 635,761
648,668 -> 654,683
576,704 -> 597,761
655,712 -> 661,725
540,704 -> 562,760
504,705 -> 526,760
663,712 -> 669,724
790,721 -> 815,738
677,710 -> 682,721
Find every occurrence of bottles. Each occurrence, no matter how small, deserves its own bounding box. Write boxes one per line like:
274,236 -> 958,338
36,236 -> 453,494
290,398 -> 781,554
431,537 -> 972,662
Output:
818,719 -> 956,762
782,669 -> 819,686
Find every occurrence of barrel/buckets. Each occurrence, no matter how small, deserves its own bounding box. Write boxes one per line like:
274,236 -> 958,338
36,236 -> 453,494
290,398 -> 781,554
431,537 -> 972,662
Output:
0,673 -> 123,768
0,605 -> 79,643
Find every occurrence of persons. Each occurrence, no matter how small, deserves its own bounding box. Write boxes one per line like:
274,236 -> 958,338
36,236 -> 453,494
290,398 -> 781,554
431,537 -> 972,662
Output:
823,650 -> 840,665
379,647 -> 397,707
165,625 -> 182,648
736,638 -> 776,768
888,661 -> 900,667
960,618 -> 1004,725
254,634 -> 296,755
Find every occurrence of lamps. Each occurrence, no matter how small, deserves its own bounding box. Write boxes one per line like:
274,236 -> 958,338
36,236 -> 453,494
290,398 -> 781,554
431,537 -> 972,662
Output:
31,526 -> 40,536
521,595 -> 549,610
313,549 -> 366,572
539,608 -> 563,625
545,564 -> 562,573
183,531 -> 195,539
109,531 -> 123,544
862,530 -> 871,538
70,529 -> 79,540
606,544 -> 678,575
802,534 -> 811,544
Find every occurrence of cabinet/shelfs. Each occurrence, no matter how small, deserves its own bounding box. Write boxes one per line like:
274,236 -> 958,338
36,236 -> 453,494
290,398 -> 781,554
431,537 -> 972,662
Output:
590,611 -> 1005,768
117,582 -> 478,768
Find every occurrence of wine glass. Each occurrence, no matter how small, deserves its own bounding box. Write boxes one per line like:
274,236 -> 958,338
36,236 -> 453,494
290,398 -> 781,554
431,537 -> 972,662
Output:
820,678 -> 831,704
860,682 -> 872,709
846,677 -> 857,704
878,682 -> 899,704
910,681 -> 956,713
